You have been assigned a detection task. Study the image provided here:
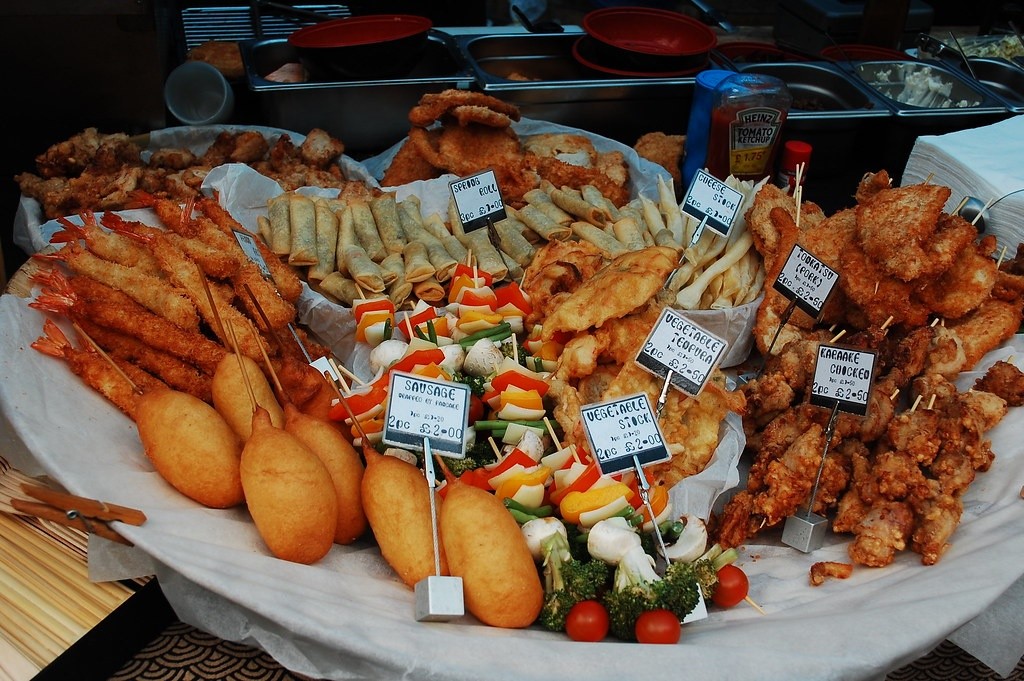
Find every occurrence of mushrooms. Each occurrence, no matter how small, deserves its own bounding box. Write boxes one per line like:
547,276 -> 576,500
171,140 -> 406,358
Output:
366,336 -> 709,567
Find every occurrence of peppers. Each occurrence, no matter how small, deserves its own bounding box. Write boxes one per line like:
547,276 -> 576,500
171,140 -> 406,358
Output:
328,263 -> 670,524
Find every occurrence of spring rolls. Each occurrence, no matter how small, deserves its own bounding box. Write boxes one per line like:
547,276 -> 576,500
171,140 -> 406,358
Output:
253,176 -> 686,313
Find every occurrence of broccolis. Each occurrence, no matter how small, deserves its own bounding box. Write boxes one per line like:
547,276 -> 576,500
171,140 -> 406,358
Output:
537,531 -> 720,640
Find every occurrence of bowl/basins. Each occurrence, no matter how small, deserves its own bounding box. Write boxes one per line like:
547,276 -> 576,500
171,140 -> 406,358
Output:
573,35 -> 712,80
819,43 -> 912,61
581,7 -> 717,74
287,14 -> 433,83
708,41 -> 815,62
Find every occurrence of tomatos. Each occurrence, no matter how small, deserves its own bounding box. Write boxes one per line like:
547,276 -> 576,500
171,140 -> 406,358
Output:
566,600 -> 609,642
710,563 -> 749,607
635,609 -> 681,644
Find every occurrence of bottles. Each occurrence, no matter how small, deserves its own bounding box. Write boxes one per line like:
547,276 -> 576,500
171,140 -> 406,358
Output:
707,73 -> 794,187
681,68 -> 738,196
777,141 -> 812,197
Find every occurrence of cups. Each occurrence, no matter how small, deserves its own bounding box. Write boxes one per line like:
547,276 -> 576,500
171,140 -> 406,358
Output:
164,62 -> 236,126
